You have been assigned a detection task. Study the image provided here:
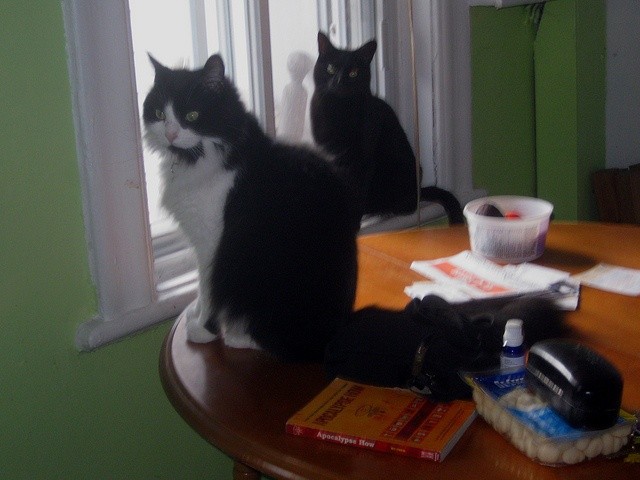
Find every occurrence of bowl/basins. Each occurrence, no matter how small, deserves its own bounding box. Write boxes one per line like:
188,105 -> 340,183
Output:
463,194 -> 554,264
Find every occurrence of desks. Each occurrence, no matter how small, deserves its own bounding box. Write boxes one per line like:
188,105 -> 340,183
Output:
158,218 -> 638,479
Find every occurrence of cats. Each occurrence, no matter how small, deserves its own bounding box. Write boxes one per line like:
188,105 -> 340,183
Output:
141,48 -> 565,362
309,29 -> 464,227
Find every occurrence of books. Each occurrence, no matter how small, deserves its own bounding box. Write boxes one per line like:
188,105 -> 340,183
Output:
285,376 -> 478,466
